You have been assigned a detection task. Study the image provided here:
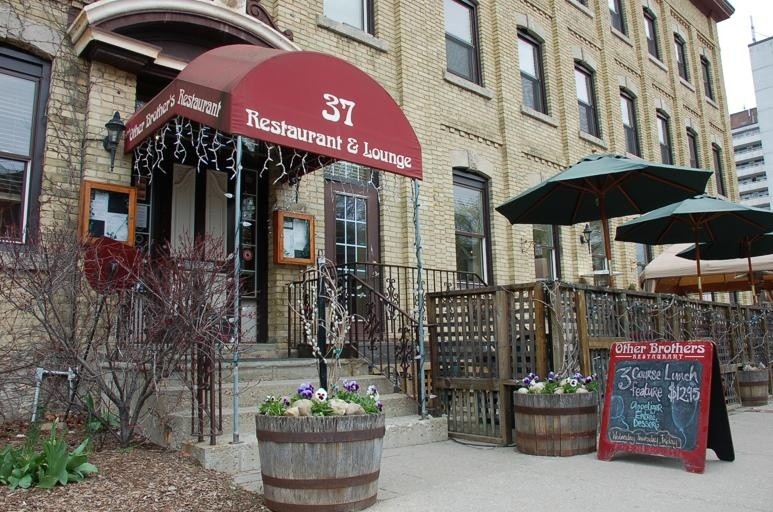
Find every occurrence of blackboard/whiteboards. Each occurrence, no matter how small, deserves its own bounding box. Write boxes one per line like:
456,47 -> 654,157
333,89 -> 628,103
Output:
595,342 -> 713,459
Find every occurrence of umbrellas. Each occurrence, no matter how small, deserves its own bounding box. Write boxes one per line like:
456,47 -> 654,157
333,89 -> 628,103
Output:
493,147 -> 714,289
675,232 -> 773,306
612,190 -> 773,303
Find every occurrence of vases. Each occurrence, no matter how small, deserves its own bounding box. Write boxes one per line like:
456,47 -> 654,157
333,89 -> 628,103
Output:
737,368 -> 769,406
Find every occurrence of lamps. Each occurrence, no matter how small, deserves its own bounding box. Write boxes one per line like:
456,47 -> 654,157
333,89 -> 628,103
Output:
580,224 -> 592,254
102,110 -> 127,172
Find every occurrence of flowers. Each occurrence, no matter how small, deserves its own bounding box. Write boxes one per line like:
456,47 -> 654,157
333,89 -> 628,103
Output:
512,368 -> 599,393
258,379 -> 383,416
